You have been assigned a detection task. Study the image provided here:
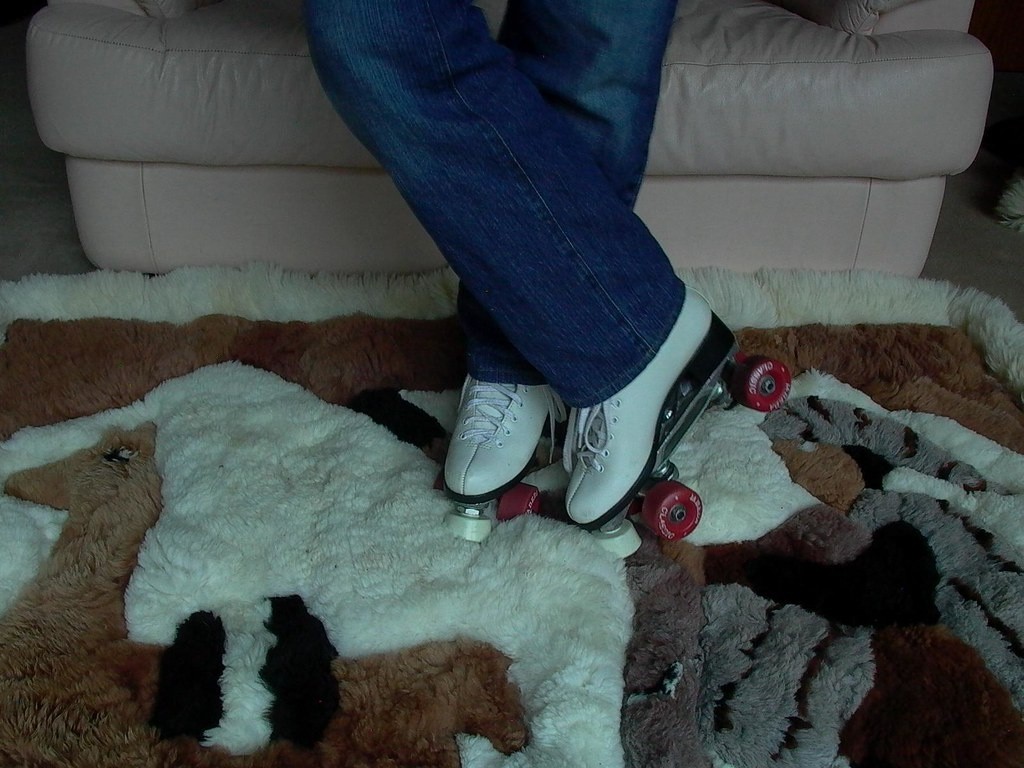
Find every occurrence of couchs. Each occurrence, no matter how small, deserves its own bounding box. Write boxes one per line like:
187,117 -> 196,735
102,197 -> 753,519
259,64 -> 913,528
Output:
23,0 -> 998,277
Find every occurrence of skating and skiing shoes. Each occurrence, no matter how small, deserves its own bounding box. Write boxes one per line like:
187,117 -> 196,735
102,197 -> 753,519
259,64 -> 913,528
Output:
445,369 -> 570,542
562,286 -> 790,560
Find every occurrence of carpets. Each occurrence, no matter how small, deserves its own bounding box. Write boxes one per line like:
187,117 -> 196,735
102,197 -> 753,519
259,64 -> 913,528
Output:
0,274 -> 1024,768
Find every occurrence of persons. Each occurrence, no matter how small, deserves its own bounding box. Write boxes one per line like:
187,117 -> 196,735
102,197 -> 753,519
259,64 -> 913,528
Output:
302,0 -> 792,545
